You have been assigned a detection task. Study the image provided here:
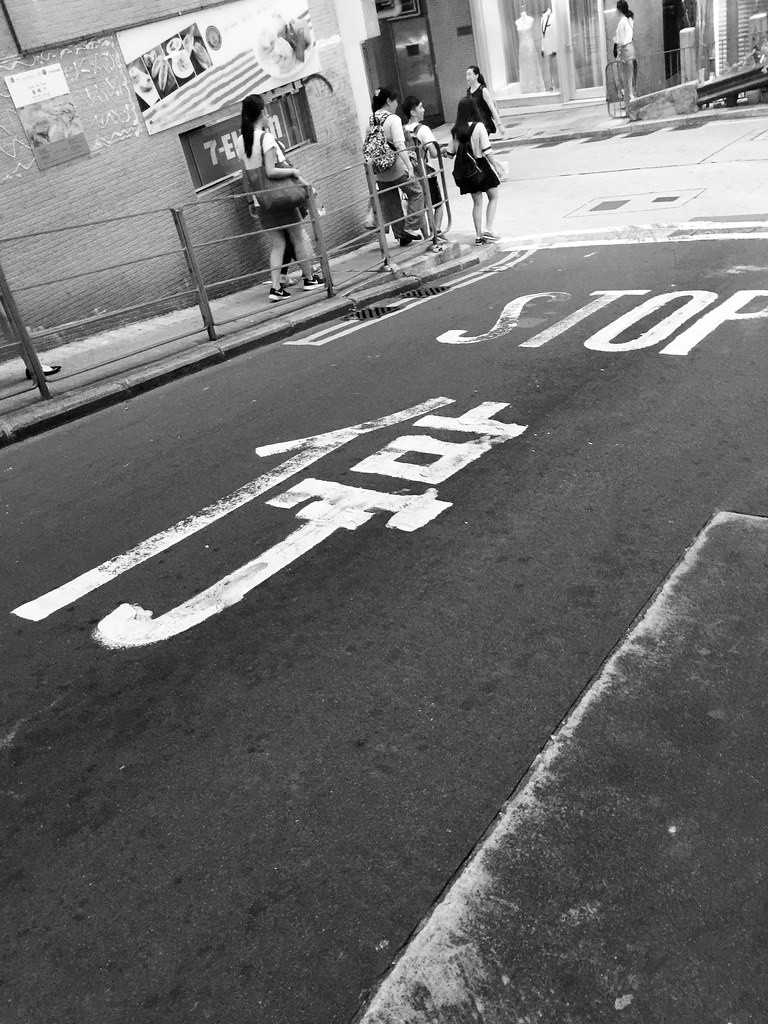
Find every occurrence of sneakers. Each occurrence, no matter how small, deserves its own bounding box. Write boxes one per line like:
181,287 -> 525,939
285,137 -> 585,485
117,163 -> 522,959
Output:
475,238 -> 484,245
268,287 -> 291,300
303,275 -> 325,291
436,229 -> 448,242
481,231 -> 500,242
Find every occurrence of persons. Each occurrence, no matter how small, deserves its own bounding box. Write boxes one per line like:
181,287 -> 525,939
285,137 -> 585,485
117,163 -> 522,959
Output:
444,96 -> 505,245
540,8 -> 559,92
465,65 -> 505,137
365,87 -> 424,247
515,12 -> 546,94
234,94 -> 324,300
402,95 -> 448,242
612,0 -> 635,102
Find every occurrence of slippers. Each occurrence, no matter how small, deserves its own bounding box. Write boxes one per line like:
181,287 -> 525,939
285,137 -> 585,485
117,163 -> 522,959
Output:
279,276 -> 299,288
302,265 -> 323,279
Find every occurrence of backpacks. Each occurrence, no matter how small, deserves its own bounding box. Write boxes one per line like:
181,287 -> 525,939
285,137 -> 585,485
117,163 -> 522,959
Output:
362,112 -> 397,175
452,121 -> 483,189
402,124 -> 429,171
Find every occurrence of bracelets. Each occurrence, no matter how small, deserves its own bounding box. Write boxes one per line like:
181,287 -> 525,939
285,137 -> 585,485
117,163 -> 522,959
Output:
497,124 -> 502,127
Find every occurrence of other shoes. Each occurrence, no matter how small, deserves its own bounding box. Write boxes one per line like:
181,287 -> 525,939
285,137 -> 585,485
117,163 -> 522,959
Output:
401,230 -> 422,240
399,238 -> 413,247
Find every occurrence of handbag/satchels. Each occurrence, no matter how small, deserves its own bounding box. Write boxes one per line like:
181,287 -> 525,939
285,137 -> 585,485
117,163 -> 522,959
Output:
245,132 -> 309,213
363,189 -> 390,234
613,43 -> 619,58
